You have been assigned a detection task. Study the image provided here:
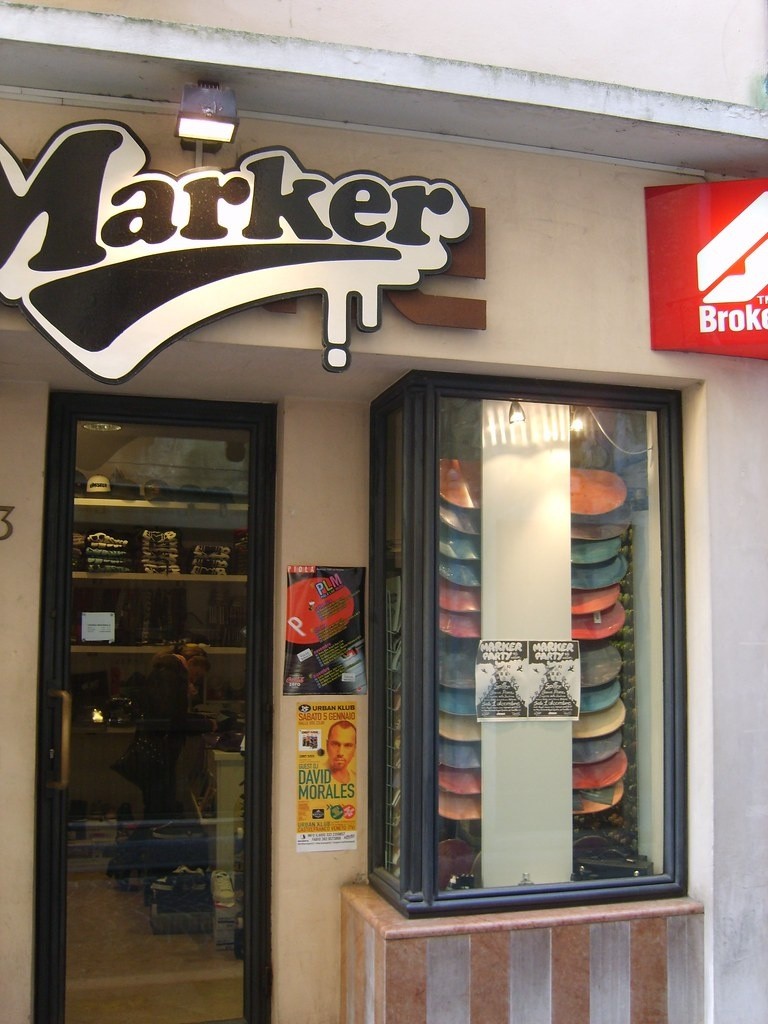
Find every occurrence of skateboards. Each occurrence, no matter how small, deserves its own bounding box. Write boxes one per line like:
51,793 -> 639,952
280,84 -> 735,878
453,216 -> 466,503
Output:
437,457 -> 636,819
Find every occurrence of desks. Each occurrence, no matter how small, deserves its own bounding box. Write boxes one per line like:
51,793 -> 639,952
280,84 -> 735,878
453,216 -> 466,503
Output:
339,886 -> 704,1024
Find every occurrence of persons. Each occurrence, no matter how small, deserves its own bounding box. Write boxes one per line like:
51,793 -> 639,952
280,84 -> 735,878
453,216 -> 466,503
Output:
323,720 -> 357,784
106,641 -> 218,893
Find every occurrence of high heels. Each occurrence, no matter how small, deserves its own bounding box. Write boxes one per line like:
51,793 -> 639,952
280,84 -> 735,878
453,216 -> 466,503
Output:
106,853 -> 139,892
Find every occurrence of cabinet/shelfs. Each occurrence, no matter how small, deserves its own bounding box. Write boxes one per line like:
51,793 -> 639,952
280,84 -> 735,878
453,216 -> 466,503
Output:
72,476 -> 250,860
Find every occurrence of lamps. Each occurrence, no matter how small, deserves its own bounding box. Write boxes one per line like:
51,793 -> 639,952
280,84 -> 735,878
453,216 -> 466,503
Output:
169,80 -> 239,146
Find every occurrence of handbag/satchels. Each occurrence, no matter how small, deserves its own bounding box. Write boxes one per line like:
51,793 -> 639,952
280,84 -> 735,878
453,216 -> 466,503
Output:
115,735 -> 160,792
